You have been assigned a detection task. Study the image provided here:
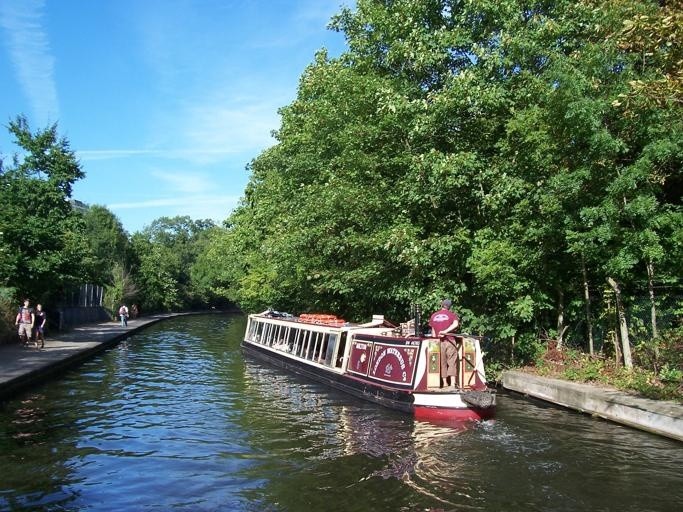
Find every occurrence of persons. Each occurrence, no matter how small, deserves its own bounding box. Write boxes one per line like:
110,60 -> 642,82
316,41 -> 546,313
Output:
33,302 -> 47,348
118,302 -> 130,329
131,302 -> 138,321
13,298 -> 36,349
428,298 -> 459,390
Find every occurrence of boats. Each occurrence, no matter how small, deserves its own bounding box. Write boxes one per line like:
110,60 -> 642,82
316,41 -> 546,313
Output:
240,302 -> 497,422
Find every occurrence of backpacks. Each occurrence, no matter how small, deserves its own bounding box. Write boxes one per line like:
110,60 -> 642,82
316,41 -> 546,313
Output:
121,308 -> 126,315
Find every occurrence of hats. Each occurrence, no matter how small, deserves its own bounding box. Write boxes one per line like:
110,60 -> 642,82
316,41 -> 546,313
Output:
441,299 -> 452,306
